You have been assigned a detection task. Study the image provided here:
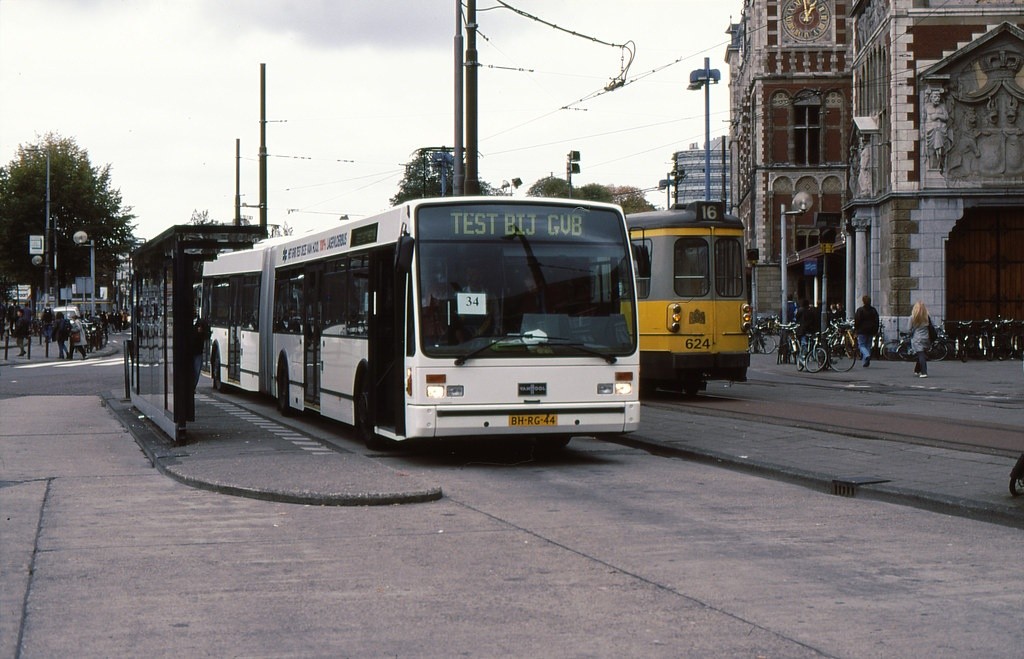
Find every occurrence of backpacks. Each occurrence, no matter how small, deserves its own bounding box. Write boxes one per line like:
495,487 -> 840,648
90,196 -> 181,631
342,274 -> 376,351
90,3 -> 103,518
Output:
60,318 -> 71,336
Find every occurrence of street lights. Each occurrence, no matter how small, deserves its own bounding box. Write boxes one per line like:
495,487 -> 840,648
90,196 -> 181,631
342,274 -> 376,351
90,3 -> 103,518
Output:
781,191 -> 814,326
689,69 -> 721,200
32,256 -> 49,309
567,150 -> 581,198
502,179 -> 522,196
73,231 -> 95,316
659,180 -> 678,211
433,152 -> 453,195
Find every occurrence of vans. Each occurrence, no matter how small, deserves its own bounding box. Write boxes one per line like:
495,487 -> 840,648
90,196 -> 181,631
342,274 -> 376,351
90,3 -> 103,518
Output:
54,306 -> 80,320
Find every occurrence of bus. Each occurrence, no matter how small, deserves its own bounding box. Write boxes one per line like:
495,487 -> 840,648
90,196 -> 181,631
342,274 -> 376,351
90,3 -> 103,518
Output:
200,196 -> 652,450
592,200 -> 752,391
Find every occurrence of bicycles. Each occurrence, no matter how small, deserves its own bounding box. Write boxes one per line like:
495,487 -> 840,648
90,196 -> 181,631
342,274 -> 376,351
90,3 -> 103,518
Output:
778,319 -> 1024,374
82,321 -> 108,352
750,315 -> 782,353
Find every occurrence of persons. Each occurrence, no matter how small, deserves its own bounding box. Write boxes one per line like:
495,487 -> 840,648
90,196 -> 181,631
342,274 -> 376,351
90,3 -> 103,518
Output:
779,294 -> 821,339
907,301 -> 935,379
829,302 -> 845,323
0,299 -> 122,360
192,304 -> 210,394
854,295 -> 879,366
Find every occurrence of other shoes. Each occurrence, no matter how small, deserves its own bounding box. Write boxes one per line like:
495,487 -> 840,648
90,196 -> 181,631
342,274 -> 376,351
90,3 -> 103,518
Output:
863,356 -> 871,367
913,370 -> 928,378
17,351 -> 26,356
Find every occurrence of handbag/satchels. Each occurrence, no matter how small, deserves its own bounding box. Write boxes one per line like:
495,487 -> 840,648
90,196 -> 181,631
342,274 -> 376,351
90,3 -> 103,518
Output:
928,315 -> 937,342
71,331 -> 80,342
